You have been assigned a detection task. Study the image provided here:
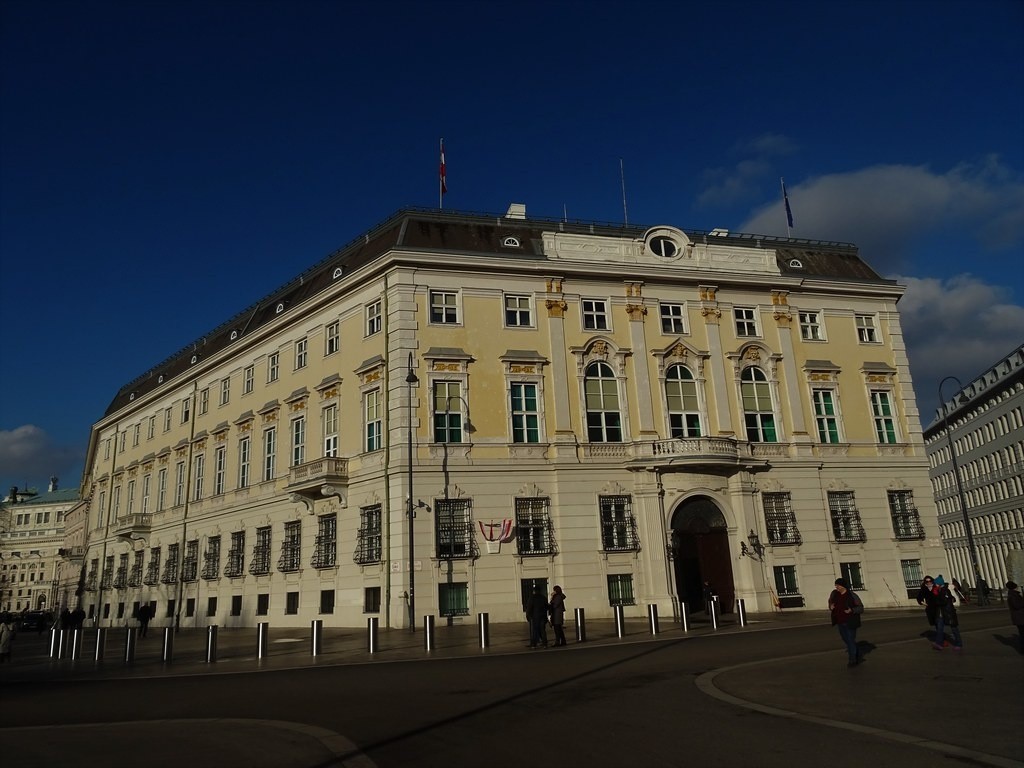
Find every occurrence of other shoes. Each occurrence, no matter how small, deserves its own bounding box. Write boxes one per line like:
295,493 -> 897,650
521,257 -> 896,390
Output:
953,646 -> 960,651
847,658 -> 858,668
932,642 -> 941,649
943,640 -> 949,647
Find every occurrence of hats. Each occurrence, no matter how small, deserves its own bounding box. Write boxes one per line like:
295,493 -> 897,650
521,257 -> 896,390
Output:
1005,581 -> 1018,589
934,575 -> 946,586
839,578 -> 849,589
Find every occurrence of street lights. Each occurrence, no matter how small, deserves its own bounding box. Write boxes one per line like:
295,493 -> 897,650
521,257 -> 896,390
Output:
404,351 -> 419,633
938,376 -> 989,608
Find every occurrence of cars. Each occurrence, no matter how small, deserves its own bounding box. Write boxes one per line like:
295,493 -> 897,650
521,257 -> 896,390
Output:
18,610 -> 54,630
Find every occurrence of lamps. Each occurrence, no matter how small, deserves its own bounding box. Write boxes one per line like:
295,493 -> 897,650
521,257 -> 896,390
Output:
741,528 -> 759,556
666,535 -> 680,561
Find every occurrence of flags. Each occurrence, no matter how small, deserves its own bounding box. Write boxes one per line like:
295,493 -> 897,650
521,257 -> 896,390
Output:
781,178 -> 793,228
440,140 -> 447,195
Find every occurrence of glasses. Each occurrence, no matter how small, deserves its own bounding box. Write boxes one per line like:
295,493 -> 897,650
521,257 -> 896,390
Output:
923,580 -> 932,584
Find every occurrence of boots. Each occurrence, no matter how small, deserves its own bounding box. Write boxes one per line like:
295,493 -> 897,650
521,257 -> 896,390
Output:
559,640 -> 567,646
552,642 -> 559,648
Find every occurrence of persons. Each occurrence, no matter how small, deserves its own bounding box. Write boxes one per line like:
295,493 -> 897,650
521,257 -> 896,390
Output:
0,611 -> 13,664
917,575 -> 990,651
51,606 -> 86,650
137,602 -> 152,638
526,584 -> 567,649
704,580 -> 712,614
1006,581 -> 1024,650
828,578 -> 864,667
